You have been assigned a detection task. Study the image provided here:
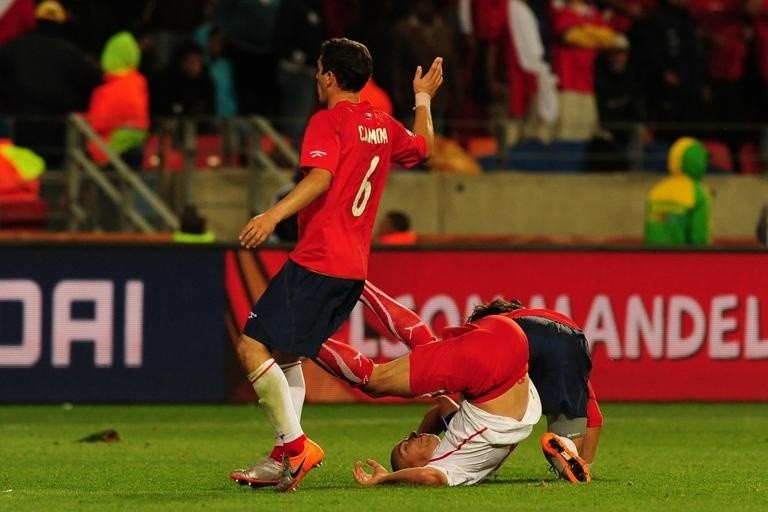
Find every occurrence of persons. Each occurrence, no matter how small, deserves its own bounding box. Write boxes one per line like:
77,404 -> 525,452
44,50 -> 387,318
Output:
417,301 -> 601,483
308,279 -> 542,486
229,37 -> 446,493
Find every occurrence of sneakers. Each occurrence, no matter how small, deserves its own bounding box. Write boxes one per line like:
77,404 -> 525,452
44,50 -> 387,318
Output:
271,438 -> 324,494
228,455 -> 289,489
538,430 -> 594,488
